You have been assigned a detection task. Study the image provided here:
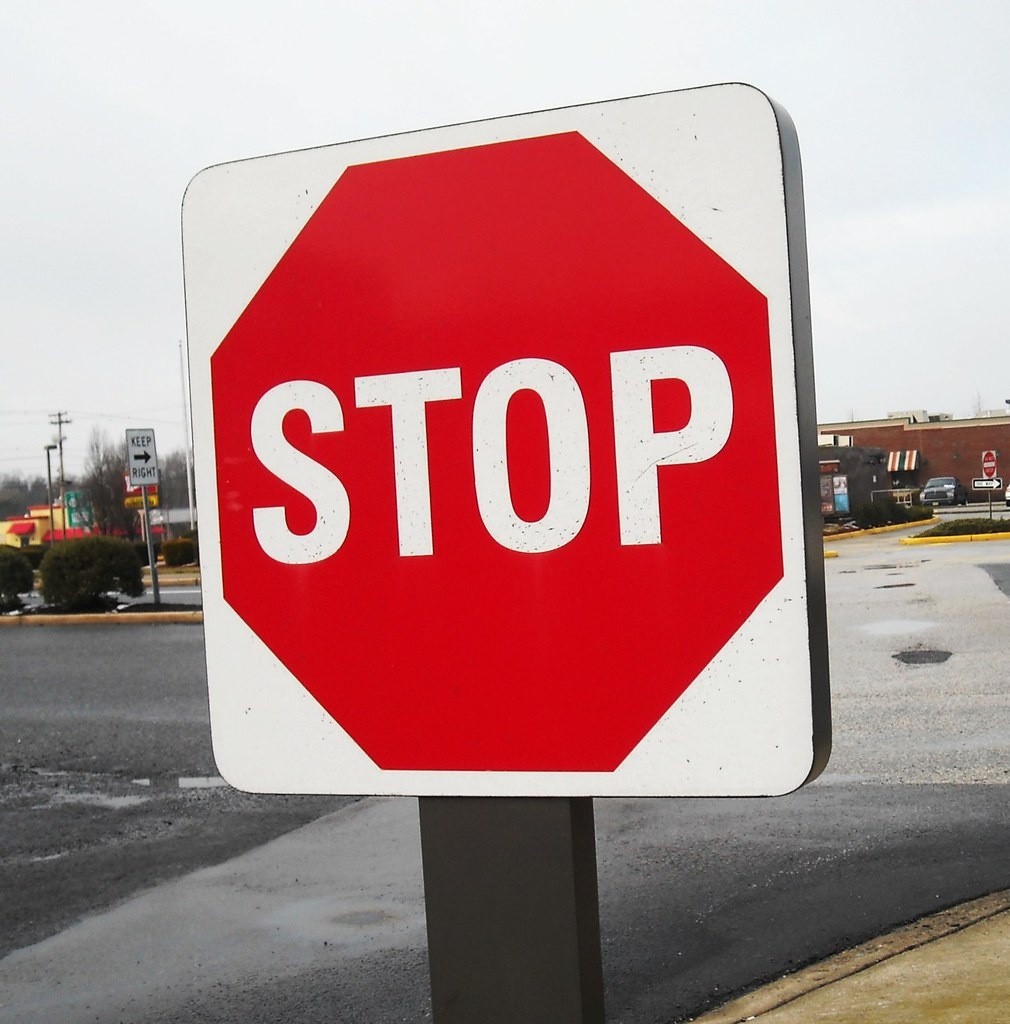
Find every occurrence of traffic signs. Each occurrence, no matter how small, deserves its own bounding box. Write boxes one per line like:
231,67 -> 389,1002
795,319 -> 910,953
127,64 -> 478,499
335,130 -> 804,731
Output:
972,476 -> 1003,490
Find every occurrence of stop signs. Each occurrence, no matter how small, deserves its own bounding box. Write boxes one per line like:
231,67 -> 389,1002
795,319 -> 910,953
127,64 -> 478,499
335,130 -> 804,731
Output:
211,124 -> 787,771
983,450 -> 996,477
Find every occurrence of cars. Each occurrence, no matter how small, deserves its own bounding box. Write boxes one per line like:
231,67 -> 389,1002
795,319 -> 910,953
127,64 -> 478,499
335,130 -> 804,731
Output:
918,476 -> 970,506
1003,484 -> 1010,508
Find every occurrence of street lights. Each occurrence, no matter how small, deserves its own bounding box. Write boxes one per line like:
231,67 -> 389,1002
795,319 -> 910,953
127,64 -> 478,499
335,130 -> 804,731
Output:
43,443 -> 59,542
